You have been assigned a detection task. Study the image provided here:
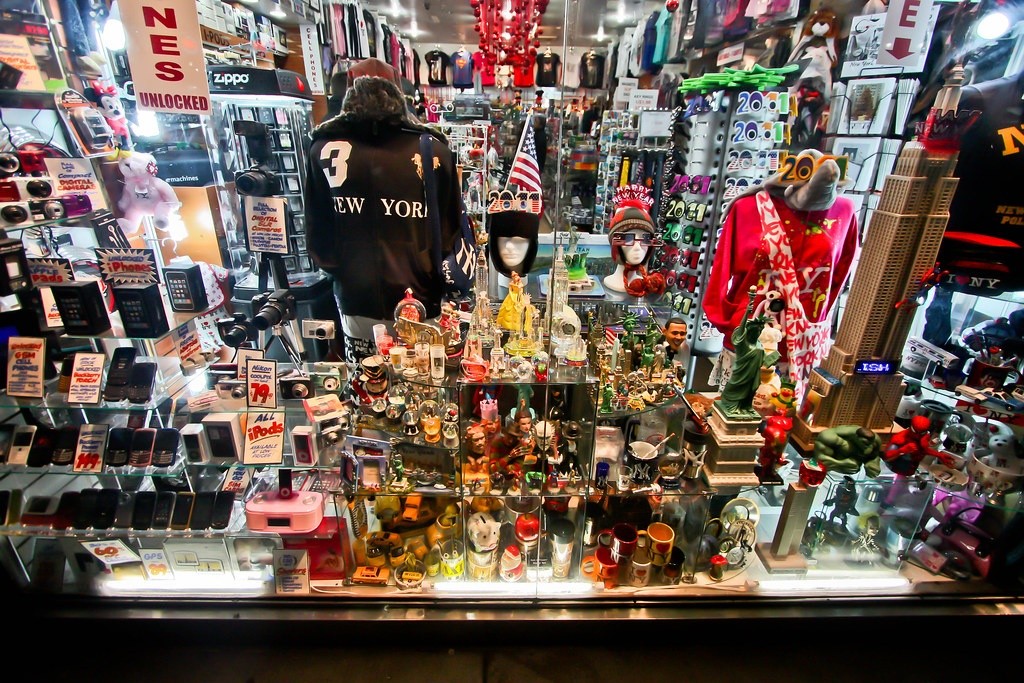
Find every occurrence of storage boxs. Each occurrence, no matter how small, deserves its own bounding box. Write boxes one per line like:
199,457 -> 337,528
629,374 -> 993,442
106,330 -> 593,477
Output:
163,263 -> 209,313
163,537 -> 235,580
179,423 -> 209,463
225,535 -> 284,583
290,426 -> 317,467
201,412 -> 244,461
112,282 -> 170,339
50,280 -> 111,338
285,515 -> 354,580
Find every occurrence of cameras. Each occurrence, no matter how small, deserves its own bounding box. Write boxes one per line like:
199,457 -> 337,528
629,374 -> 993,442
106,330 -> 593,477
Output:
233,119 -> 281,197
280,362 -> 347,399
251,289 -> 298,331
302,319 -> 335,340
207,362 -> 247,399
217,312 -> 260,347
317,425 -> 344,448
0,151 -> 92,228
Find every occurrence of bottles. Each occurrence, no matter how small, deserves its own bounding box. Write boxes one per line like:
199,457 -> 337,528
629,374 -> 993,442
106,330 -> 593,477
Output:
549,519 -> 575,578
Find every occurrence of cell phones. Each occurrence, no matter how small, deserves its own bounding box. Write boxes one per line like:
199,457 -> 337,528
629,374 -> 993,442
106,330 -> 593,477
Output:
165,271 -> 194,310
0,347 -> 234,531
61,289 -> 90,331
119,292 -> 153,335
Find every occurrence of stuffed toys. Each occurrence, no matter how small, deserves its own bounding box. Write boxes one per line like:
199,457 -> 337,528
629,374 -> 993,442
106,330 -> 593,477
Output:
115,152 -> 181,235
980,434 -> 1024,475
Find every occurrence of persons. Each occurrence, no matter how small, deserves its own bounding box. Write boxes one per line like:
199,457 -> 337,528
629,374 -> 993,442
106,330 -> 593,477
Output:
487,208 -> 539,278
700,148 -> 858,393
662,318 -> 687,361
815,424 -> 882,478
414,93 -> 428,123
604,200 -> 655,292
722,301 -> 777,414
463,383 -> 584,488
852,511 -> 880,566
823,485 -> 859,533
321,71 -> 348,124
304,59 -> 476,430
885,416 -> 955,476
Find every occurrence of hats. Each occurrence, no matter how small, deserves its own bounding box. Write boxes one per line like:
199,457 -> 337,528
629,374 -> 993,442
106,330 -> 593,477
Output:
607,182 -> 657,246
487,190 -> 543,280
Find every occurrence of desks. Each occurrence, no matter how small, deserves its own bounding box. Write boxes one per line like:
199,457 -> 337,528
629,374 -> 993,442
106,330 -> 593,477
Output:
490,267 -> 634,334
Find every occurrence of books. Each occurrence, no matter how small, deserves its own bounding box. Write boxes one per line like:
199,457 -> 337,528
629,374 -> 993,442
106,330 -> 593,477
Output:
762,351 -> 781,370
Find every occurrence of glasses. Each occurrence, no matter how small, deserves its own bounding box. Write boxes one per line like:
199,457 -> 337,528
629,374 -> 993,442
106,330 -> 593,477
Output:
612,232 -> 665,247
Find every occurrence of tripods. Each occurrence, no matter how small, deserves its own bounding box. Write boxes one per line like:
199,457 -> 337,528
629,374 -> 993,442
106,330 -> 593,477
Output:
263,324 -> 305,378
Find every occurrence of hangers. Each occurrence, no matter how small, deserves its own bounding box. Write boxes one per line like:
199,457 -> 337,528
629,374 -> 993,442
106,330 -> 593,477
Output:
328,0 -> 595,56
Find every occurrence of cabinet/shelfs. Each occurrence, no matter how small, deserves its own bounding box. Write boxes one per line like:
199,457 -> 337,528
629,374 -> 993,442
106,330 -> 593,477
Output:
895,292 -> 1024,418
335,346 -> 719,586
0,0 -> 287,606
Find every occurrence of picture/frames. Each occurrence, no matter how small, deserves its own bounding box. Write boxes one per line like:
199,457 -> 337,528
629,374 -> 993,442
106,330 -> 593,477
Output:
309,0 -> 320,12
294,0 -> 304,17
304,3 -> 316,24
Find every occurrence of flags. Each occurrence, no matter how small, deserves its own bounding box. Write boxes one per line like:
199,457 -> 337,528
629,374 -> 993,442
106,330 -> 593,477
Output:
509,117 -> 543,203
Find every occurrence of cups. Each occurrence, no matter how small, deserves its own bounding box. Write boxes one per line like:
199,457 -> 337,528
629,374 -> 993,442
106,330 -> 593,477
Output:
597,522 -> 639,565
654,546 -> 685,585
622,440 -> 659,488
965,355 -> 1023,395
580,548 -> 620,589
637,522 -> 674,566
625,545 -> 653,587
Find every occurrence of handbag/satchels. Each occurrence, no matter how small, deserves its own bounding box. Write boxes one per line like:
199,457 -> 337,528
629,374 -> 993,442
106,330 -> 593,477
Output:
756,190 -> 835,406
419,132 -> 477,300
